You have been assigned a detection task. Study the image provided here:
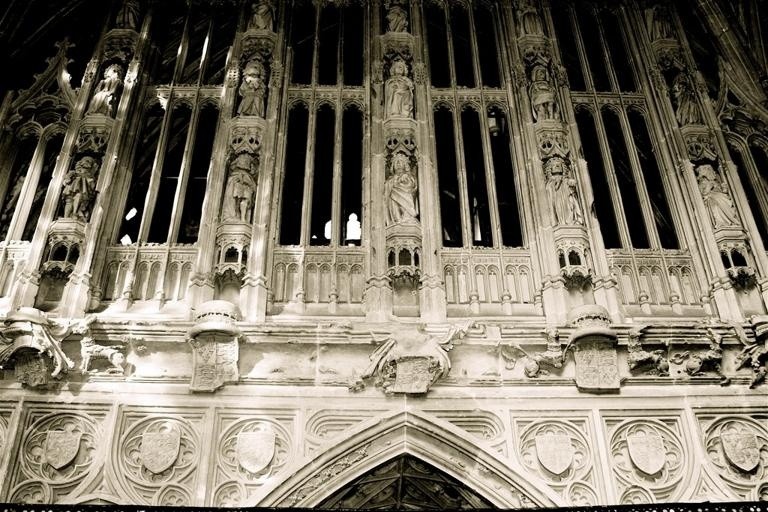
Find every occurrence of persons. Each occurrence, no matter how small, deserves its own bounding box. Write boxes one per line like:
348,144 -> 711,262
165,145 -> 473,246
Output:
56,0 -> 141,221
642,1 -> 742,230
513,1 -> 585,229
220,0 -> 278,224
381,1 -> 420,227
